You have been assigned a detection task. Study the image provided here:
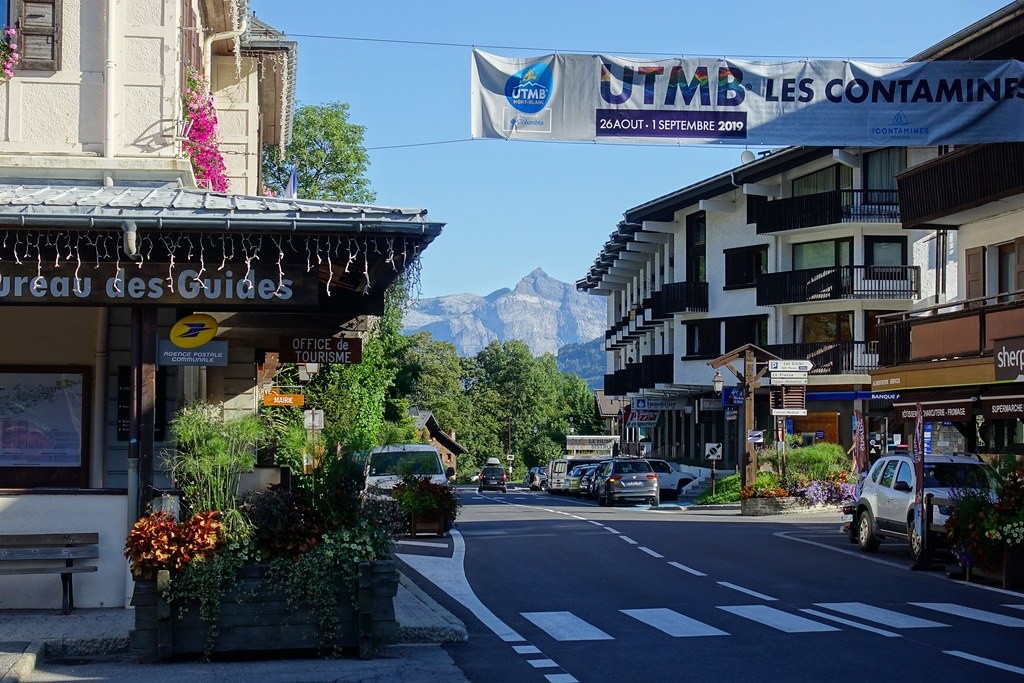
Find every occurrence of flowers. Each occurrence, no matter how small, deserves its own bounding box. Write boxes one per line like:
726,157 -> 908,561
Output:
122,507 -> 227,577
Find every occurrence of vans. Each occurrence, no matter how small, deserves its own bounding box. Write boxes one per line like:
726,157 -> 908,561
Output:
361,444 -> 455,504
548,457 -> 605,491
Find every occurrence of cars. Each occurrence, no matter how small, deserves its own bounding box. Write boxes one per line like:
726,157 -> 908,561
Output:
478,465 -> 509,494
528,467 -> 547,491
566,459 -> 698,508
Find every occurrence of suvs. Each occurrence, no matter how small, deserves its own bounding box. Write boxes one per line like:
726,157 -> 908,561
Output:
852,452 -> 1009,562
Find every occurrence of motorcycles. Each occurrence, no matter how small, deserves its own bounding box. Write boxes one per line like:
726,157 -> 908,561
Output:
842,494 -> 858,543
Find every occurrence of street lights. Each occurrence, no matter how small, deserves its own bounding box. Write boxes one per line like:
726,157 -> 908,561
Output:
707,344 -> 785,488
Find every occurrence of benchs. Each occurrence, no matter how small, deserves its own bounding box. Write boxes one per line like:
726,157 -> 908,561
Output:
0,532 -> 100,614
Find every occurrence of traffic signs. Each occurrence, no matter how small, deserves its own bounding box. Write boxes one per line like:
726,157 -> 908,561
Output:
768,359 -> 816,372
